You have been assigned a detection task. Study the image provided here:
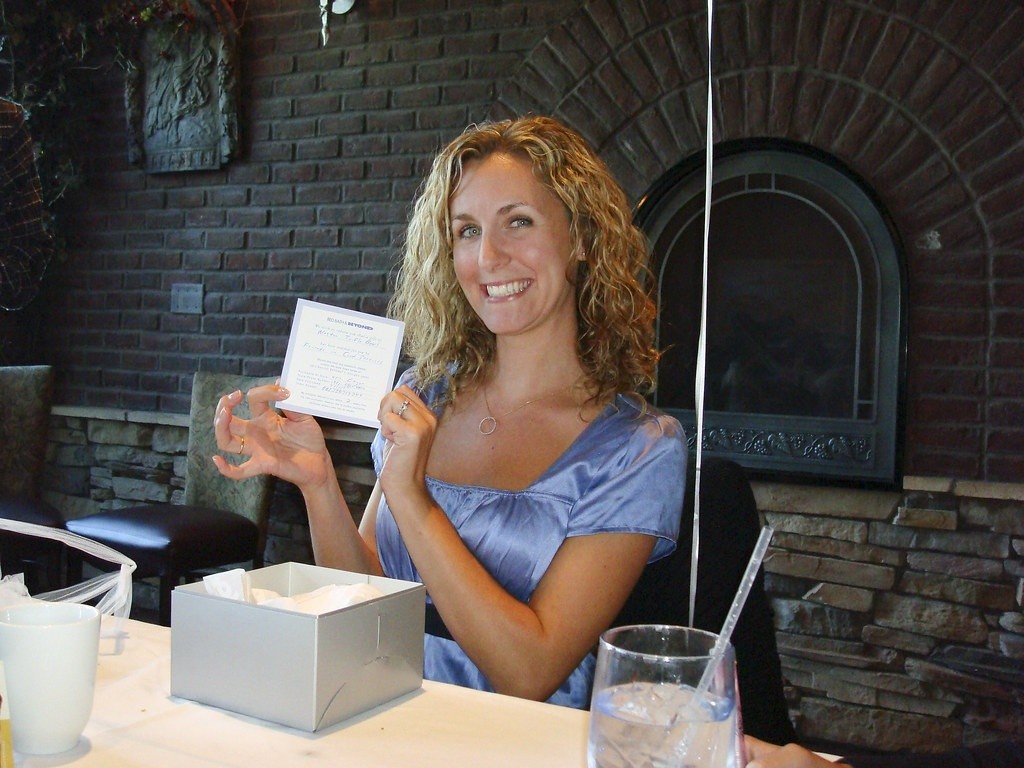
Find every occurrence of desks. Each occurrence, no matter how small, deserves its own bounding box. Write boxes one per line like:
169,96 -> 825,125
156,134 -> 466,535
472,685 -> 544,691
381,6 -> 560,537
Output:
0,613 -> 852,768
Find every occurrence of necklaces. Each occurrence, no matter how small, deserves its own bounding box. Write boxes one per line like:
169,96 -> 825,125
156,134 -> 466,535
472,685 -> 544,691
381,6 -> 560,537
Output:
478,369 -> 586,435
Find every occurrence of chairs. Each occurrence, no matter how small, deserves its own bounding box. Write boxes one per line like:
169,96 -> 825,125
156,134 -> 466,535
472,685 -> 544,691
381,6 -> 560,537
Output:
67,370 -> 281,627
614,453 -> 795,749
0,365 -> 66,592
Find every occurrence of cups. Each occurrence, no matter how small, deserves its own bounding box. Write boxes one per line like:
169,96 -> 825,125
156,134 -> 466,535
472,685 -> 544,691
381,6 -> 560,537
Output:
585,623 -> 746,768
1,603 -> 101,757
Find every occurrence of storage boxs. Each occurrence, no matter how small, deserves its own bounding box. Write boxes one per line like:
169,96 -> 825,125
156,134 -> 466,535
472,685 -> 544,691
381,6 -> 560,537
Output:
170,562 -> 425,735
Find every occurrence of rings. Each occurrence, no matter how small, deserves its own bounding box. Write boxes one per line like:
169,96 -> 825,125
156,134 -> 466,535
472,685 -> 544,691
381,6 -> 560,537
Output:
236,436 -> 245,454
398,399 -> 410,418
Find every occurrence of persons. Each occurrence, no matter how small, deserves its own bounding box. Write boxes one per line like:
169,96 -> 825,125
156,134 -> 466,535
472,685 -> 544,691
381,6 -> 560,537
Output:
211,116 -> 689,710
